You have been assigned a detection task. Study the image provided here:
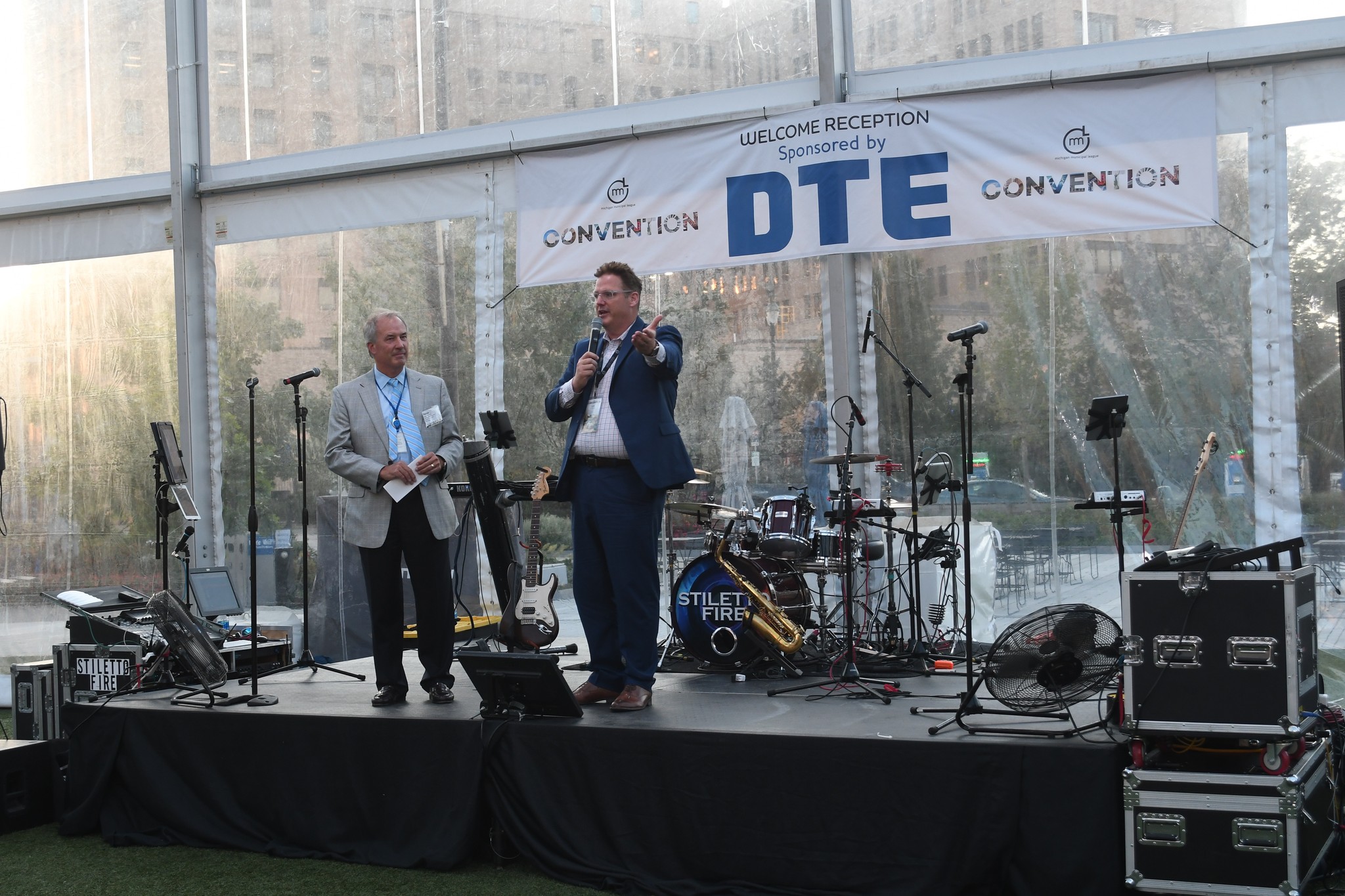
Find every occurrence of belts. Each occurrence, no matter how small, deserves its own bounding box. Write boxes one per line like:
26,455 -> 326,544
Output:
576,455 -> 631,468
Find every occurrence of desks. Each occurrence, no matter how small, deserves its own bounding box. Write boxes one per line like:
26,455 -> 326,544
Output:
1312,539 -> 1345,603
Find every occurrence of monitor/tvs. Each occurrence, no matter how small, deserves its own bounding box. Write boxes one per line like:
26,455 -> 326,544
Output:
187,565 -> 244,617
457,651 -> 585,717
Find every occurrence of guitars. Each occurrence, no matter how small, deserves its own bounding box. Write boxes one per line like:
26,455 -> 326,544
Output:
1168,432 -> 1216,547
499,466 -> 560,647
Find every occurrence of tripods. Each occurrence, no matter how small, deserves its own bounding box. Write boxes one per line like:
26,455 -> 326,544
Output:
767,331 -> 1076,735
235,378 -> 366,685
653,494 -> 687,668
89,448 -> 227,702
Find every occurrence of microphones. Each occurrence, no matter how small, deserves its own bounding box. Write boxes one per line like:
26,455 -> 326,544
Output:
862,311 -> 872,352
588,317 -> 604,359
283,368 -> 320,385
172,526 -> 195,556
829,517 -> 837,528
847,396 -> 866,426
947,320 -> 989,342
910,451 -> 923,483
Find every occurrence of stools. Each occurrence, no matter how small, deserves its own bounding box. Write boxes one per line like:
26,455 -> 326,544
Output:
833,539 -> 888,636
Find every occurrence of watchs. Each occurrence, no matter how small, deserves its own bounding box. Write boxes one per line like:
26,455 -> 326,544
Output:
648,339 -> 660,356
441,457 -> 447,475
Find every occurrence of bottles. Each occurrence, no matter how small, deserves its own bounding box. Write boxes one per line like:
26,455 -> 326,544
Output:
216,614 -> 230,632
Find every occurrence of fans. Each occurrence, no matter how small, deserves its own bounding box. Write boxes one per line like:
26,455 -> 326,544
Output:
954,603 -> 1123,738
146,589 -> 229,710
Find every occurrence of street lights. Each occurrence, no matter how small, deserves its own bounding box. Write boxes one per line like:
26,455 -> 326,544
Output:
764,295 -> 782,437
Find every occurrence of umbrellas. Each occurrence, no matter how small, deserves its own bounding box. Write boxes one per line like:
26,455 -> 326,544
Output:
799,392 -> 832,529
719,390 -> 759,536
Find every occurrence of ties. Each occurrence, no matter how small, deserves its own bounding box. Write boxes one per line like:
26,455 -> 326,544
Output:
388,379 -> 431,486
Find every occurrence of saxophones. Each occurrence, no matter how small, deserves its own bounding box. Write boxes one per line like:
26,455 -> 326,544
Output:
714,519 -> 806,654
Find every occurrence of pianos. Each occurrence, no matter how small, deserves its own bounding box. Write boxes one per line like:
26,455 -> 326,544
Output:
448,478 -> 558,651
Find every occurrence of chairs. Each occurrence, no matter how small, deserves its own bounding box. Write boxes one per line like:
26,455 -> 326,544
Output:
995,528 -> 1099,615
1319,541 -> 1345,611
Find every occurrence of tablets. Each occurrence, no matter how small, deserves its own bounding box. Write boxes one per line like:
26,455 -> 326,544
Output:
170,485 -> 201,520
158,421 -> 188,483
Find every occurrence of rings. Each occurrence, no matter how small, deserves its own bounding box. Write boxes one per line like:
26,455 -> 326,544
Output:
431,464 -> 434,470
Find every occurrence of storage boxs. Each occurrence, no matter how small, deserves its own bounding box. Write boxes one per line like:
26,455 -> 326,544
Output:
37,668 -> 56,741
1120,565 -> 1322,744
52,639 -> 294,739
1121,729 -> 1339,896
10,659 -> 53,740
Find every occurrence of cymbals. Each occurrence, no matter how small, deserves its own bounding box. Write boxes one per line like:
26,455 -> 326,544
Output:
808,453 -> 890,464
665,502 -> 754,520
686,478 -> 710,484
693,468 -> 713,475
883,498 -> 913,509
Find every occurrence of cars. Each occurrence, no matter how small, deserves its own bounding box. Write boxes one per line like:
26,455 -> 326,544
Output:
937,478 -> 1086,504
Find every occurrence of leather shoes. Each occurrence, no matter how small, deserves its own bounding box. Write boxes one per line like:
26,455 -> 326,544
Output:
572,680 -> 620,705
610,684 -> 653,711
371,686 -> 406,706
429,682 -> 454,704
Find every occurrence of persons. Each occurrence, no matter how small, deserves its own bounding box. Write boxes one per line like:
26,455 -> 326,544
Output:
544,261 -> 697,709
324,308 -> 464,706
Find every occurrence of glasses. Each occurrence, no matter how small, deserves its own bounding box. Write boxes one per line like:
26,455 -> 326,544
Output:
593,290 -> 633,301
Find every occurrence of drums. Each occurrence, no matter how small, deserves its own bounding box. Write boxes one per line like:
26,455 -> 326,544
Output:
795,529 -> 860,573
669,551 -> 812,667
738,532 -> 766,557
756,493 -> 816,561
702,530 -> 739,553
